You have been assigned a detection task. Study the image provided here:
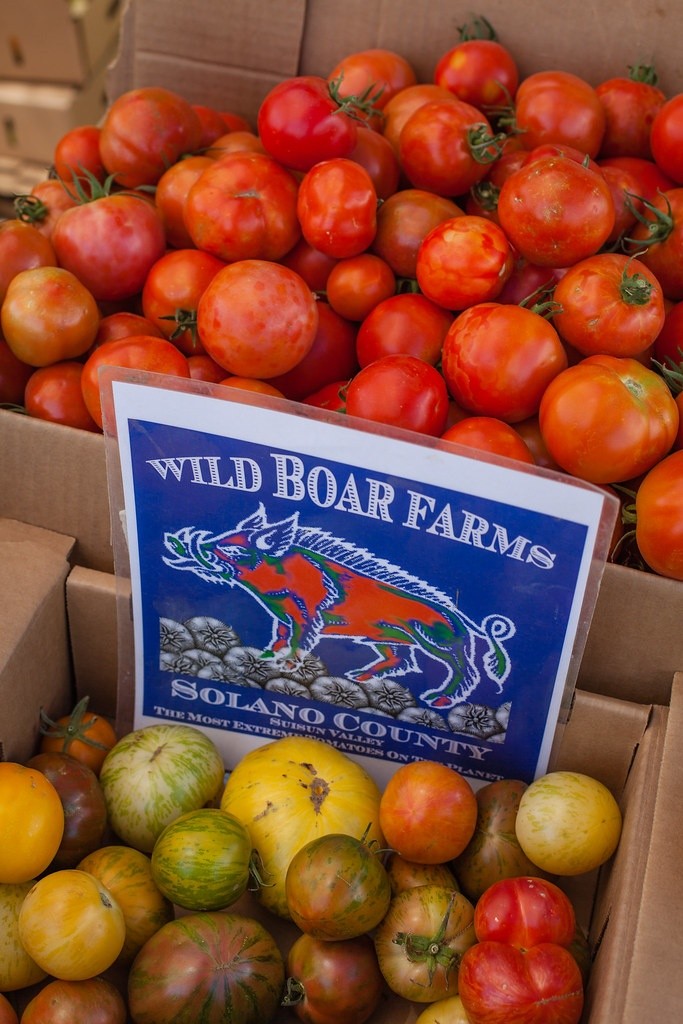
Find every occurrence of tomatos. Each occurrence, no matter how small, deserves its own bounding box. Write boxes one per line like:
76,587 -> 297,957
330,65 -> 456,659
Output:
0,694 -> 624,1023
1,16 -> 682,583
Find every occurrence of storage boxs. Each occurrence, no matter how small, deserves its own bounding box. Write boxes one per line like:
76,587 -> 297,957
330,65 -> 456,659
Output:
0,1 -> 682,222
1,410 -> 683,1024
